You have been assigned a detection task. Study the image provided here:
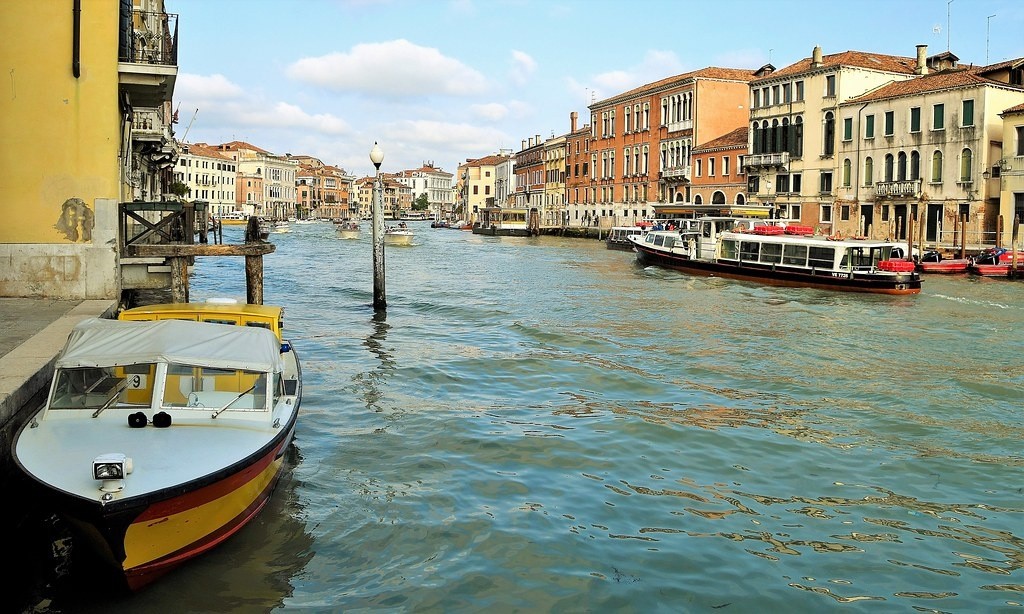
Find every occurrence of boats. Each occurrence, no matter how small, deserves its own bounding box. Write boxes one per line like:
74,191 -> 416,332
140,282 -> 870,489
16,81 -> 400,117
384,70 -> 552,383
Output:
384,223 -> 417,246
335,222 -> 362,240
4,301 -> 303,605
449,220 -> 472,229
627,216 -> 925,295
369,222 -> 386,236
471,206 -> 528,237
916,245 -> 1024,278
256,215 -> 329,234
605,221 -> 655,251
333,217 -> 343,224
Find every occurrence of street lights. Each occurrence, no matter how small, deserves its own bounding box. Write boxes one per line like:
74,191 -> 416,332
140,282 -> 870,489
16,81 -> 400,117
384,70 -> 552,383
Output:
369,141 -> 389,309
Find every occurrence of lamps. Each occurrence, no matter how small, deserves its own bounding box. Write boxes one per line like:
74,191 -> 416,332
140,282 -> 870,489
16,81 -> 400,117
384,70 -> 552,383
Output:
983,167 -> 991,180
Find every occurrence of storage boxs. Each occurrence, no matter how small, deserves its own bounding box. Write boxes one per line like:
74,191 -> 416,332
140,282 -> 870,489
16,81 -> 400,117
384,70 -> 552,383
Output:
878,261 -> 915,272
786,225 -> 814,234
753,226 -> 783,236
